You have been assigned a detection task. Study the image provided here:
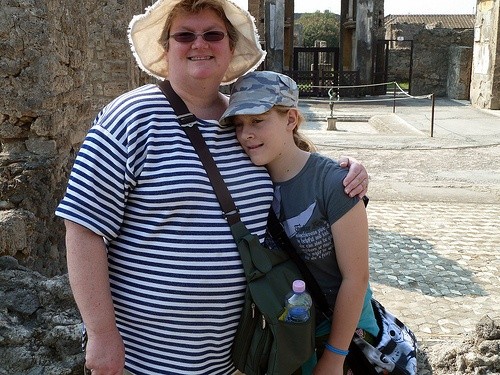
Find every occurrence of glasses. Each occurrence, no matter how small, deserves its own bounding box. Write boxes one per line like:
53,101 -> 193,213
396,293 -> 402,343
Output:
168,31 -> 228,43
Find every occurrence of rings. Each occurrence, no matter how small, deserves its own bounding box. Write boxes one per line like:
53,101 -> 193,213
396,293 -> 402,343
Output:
361,183 -> 367,189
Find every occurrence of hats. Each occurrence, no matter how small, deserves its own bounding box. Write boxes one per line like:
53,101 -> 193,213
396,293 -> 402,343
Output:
219,71 -> 299,125
127,0 -> 267,86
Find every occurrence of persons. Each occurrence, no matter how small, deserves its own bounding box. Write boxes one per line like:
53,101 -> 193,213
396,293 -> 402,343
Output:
55,1 -> 368,375
220,71 -> 379,375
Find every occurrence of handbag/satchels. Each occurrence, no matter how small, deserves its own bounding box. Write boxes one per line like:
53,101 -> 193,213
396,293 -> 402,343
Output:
347,298 -> 418,375
232,235 -> 316,375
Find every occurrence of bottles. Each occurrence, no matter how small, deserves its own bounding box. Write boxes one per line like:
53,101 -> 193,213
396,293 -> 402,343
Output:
285,280 -> 312,322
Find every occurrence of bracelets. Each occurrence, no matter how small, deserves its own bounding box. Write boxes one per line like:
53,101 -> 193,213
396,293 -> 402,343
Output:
326,344 -> 348,356
